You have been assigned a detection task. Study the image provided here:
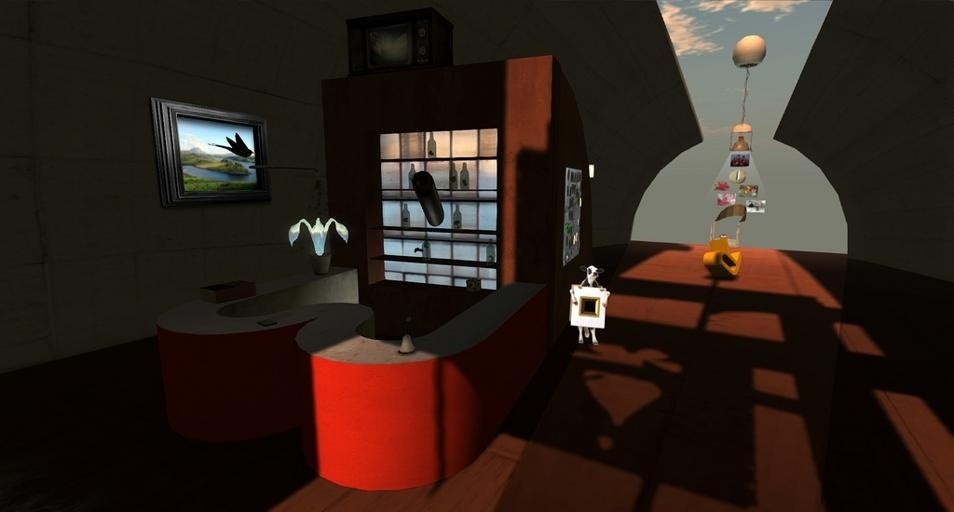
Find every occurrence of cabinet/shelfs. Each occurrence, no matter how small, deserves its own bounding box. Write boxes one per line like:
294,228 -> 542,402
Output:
373,125 -> 501,294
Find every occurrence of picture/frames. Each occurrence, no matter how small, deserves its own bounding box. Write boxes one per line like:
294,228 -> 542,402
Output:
149,98 -> 269,207
